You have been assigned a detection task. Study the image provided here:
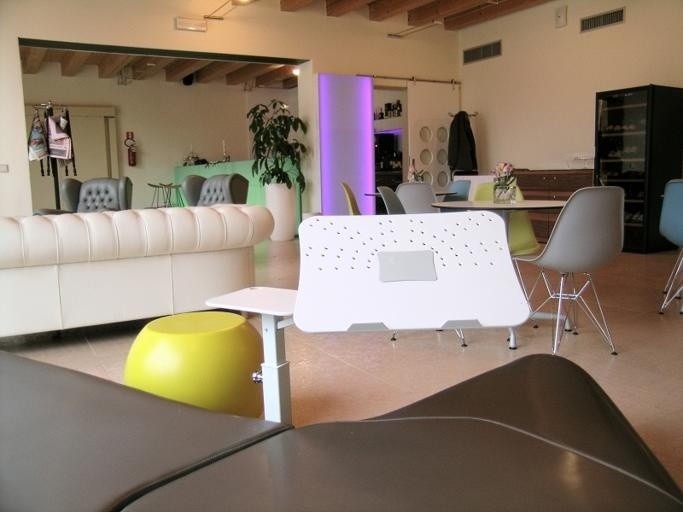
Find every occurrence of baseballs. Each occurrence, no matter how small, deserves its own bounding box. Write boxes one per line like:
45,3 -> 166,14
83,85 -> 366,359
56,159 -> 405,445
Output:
125,138 -> 137,166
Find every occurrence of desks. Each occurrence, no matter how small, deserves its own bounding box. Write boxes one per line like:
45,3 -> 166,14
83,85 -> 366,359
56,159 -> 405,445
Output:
207,285 -> 308,424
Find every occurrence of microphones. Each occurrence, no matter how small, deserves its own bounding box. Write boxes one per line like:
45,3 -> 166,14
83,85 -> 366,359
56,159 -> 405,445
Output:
147,181 -> 183,206
119,310 -> 266,416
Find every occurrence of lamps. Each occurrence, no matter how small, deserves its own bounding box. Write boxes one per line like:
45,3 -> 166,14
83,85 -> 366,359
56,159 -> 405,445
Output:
653,177 -> 683,316
34,175 -> 134,215
182,172 -> 249,206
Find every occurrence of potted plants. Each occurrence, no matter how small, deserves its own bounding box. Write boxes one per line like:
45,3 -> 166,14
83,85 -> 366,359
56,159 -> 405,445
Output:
248,98 -> 310,242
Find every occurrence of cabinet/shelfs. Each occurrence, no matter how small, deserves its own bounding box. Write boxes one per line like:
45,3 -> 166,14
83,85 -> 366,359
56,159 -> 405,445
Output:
594,88 -> 680,254
374,99 -> 402,120
374,143 -> 401,170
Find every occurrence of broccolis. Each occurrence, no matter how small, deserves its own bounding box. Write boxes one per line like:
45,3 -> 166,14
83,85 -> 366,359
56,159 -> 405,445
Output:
593,84 -> 683,255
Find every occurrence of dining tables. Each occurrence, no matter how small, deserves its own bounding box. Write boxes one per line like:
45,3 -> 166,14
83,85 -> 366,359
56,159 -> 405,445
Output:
248,98 -> 310,242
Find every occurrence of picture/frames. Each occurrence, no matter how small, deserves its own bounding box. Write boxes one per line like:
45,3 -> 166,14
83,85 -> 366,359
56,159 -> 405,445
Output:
207,285 -> 308,424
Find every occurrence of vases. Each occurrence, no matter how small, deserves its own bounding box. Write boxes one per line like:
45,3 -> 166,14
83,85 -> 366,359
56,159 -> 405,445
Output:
491,162 -> 514,196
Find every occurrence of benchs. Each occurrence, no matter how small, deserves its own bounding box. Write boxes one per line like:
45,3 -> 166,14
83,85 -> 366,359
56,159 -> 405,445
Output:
294,210 -> 525,332
1,347 -> 680,510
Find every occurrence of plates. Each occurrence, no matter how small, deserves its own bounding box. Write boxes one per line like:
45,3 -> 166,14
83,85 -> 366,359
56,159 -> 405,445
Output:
594,88 -> 680,254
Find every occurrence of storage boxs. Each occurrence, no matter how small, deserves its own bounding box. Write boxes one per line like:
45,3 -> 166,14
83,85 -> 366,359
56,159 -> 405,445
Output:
294,210 -> 525,332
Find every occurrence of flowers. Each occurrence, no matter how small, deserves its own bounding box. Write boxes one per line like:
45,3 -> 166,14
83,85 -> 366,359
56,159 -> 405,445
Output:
491,162 -> 514,196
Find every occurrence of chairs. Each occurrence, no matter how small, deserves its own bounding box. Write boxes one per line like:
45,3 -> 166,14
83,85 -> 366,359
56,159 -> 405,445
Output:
34,175 -> 134,215
182,172 -> 249,206
653,177 -> 683,316
341,176 -> 572,331
507,186 -> 625,354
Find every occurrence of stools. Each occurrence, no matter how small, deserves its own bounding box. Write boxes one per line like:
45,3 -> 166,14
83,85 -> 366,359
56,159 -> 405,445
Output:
147,181 -> 183,206
119,310 -> 266,416
